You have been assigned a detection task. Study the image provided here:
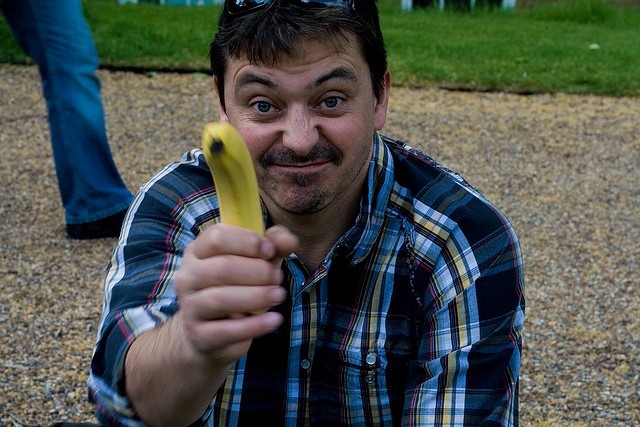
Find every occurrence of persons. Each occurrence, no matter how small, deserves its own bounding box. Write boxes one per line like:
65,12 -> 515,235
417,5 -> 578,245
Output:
0,0 -> 136,241
86,0 -> 526,426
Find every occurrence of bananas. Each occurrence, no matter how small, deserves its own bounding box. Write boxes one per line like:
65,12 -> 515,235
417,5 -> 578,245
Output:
201,121 -> 265,239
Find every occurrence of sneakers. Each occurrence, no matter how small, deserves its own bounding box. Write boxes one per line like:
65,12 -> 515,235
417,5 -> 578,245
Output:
65,208 -> 130,239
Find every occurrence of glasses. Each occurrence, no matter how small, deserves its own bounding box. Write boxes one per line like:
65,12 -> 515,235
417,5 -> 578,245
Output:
223,0 -> 361,20
